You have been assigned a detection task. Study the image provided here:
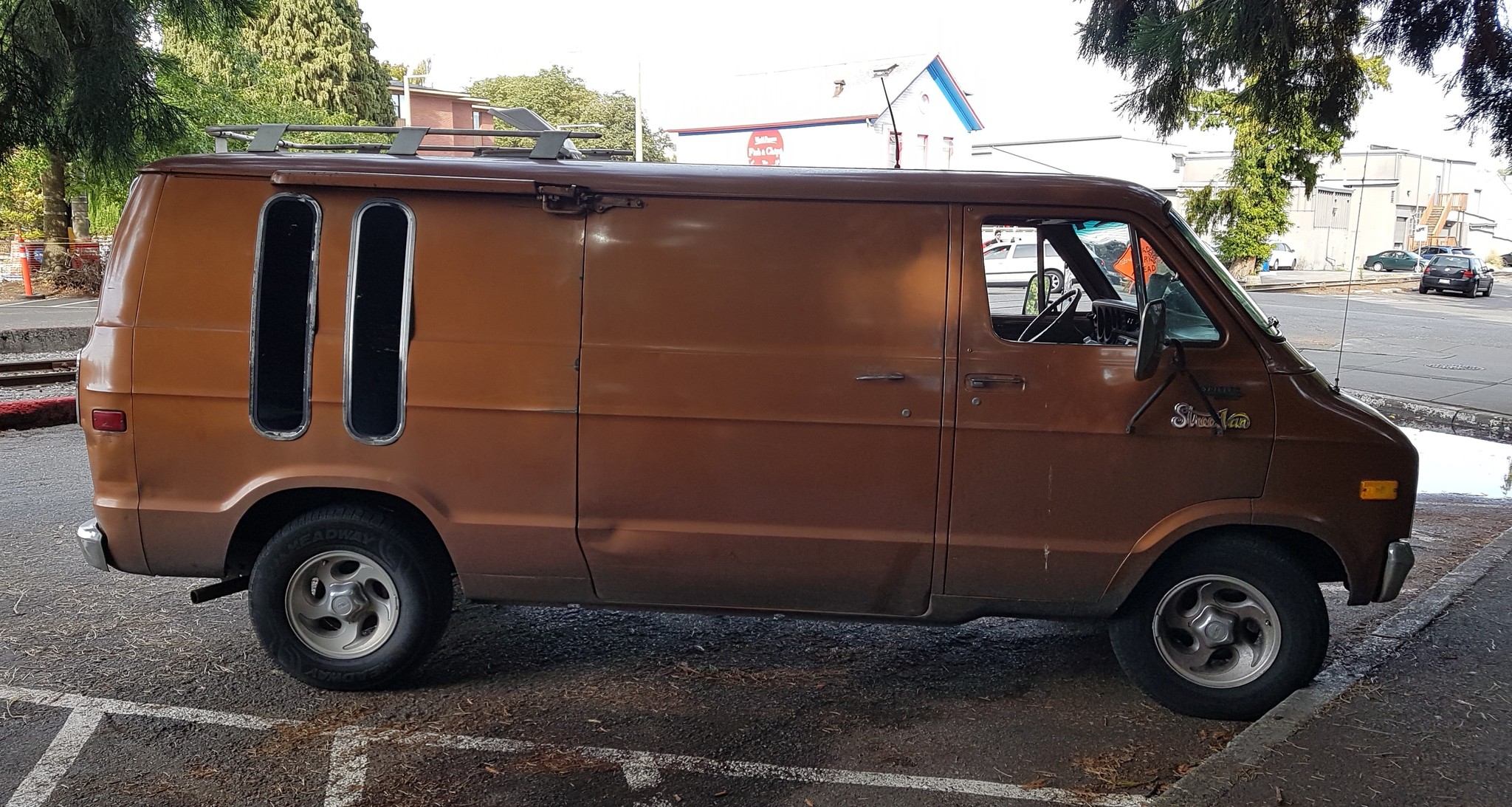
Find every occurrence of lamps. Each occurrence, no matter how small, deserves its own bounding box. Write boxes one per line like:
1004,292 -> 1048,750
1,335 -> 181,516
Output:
834,80 -> 845,87
1446,223 -> 1452,227
1407,191 -> 1412,195
1333,208 -> 1338,214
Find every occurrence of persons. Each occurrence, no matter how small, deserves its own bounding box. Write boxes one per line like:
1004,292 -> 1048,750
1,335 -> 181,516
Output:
989,230 -> 1004,252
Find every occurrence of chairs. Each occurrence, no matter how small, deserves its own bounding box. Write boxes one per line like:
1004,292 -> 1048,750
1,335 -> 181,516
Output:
1449,261 -> 1458,266
1461,262 -> 1468,267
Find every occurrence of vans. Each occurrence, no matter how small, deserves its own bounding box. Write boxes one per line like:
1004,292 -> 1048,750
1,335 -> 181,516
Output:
71,106 -> 1421,722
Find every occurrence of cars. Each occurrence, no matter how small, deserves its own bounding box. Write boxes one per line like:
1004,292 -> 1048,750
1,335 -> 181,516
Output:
1419,252 -> 1494,299
1411,245 -> 1476,260
1365,250 -> 1431,272
1268,241 -> 1297,271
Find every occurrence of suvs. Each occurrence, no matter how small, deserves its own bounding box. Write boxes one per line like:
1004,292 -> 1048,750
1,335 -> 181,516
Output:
982,236 -> 1108,293
